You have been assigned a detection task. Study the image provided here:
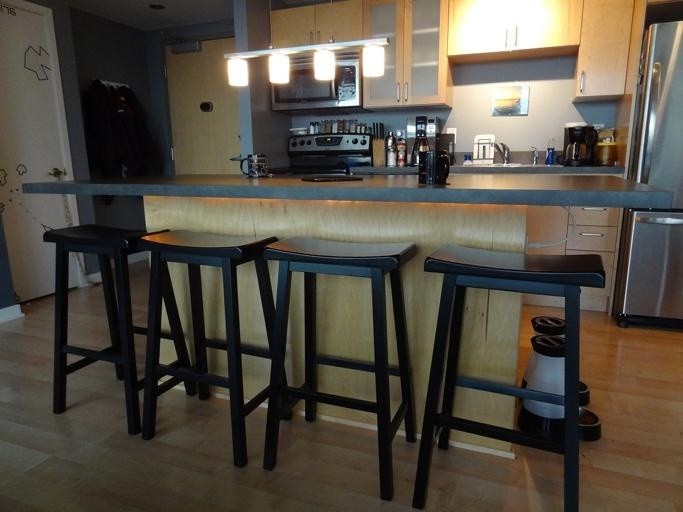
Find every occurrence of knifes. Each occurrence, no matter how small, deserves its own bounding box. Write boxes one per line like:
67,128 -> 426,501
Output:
373,121 -> 385,140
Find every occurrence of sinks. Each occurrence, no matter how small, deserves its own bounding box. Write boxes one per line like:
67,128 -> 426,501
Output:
492,162 -> 520,167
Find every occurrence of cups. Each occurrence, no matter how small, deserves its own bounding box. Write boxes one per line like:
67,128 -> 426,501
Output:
418,150 -> 450,184
239,154 -> 268,178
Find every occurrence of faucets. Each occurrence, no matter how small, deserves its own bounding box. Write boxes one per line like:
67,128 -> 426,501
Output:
530,147 -> 539,165
490,140 -> 512,163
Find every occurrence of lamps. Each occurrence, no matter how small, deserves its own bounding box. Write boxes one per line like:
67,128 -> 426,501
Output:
224,0 -> 391,88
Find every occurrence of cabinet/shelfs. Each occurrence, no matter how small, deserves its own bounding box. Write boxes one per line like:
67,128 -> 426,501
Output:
362,0 -> 454,112
448,0 -> 584,64
573,0 -> 634,102
521,205 -> 624,314
270,0 -> 363,48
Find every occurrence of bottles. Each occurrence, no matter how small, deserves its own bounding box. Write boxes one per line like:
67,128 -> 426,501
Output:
387,129 -> 406,167
545,137 -> 557,166
309,119 -> 372,135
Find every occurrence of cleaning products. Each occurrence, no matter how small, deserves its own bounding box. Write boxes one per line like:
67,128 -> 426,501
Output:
544,136 -> 555,164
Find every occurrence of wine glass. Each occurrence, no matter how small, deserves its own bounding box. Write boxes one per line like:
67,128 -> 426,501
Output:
595,142 -> 617,166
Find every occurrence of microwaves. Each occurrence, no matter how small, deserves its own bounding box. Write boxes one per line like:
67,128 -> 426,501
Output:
271,57 -> 365,113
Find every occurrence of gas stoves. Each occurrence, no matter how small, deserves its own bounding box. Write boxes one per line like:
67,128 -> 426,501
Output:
271,160 -> 372,176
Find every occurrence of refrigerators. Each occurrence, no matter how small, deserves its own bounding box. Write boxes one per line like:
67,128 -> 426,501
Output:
612,21 -> 683,327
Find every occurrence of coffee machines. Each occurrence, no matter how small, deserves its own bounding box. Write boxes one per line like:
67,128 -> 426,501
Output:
561,122 -> 596,167
407,114 -> 440,166
